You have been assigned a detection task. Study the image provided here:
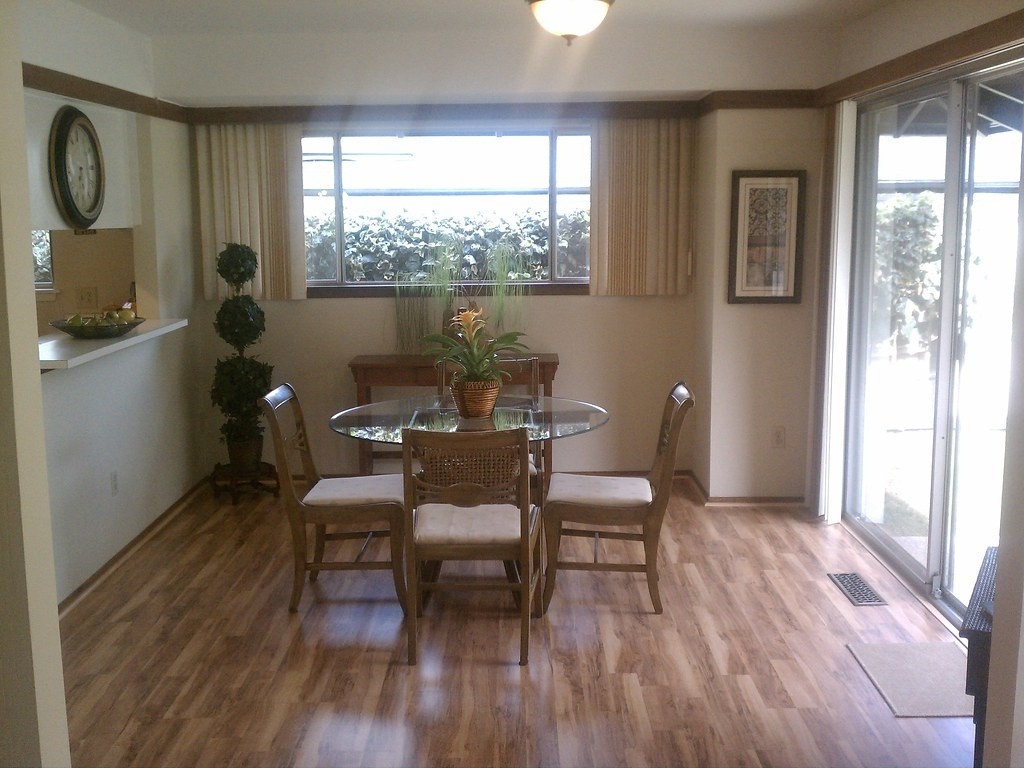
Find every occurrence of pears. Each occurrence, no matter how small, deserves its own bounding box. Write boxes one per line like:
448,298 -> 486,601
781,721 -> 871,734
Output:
66,310 -> 137,337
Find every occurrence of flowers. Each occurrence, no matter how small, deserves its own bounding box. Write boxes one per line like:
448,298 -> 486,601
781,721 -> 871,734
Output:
419,306 -> 531,387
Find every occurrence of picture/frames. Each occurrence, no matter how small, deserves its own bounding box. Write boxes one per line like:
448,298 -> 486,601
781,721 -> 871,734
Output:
726,167 -> 806,305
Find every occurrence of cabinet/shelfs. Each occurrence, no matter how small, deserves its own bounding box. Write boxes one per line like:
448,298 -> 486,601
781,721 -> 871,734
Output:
959,544 -> 999,766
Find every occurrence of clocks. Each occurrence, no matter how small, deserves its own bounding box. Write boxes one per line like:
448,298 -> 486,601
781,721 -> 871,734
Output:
49,104 -> 106,229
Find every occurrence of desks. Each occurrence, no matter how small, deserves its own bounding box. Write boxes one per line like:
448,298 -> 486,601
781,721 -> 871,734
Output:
328,389 -> 610,615
348,352 -> 560,477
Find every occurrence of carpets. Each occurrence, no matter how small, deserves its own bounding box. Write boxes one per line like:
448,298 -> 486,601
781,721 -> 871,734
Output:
846,639 -> 975,717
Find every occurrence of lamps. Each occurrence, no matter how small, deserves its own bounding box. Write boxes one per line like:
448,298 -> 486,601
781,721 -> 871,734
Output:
525,0 -> 615,46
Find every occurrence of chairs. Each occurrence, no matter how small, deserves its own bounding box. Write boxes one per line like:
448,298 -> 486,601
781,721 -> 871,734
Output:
401,427 -> 542,667
536,380 -> 695,617
443,358 -> 545,508
256,382 -> 418,617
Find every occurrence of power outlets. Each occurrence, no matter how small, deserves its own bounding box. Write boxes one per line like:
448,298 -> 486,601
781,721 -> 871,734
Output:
80,286 -> 99,309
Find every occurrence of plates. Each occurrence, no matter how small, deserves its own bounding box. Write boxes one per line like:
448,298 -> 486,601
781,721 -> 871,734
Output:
47,315 -> 148,340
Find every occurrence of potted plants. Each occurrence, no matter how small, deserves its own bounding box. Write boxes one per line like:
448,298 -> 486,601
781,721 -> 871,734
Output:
208,241 -> 277,474
392,233 -> 534,348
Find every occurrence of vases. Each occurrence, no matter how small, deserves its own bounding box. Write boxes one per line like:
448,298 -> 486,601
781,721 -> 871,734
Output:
447,376 -> 501,418
